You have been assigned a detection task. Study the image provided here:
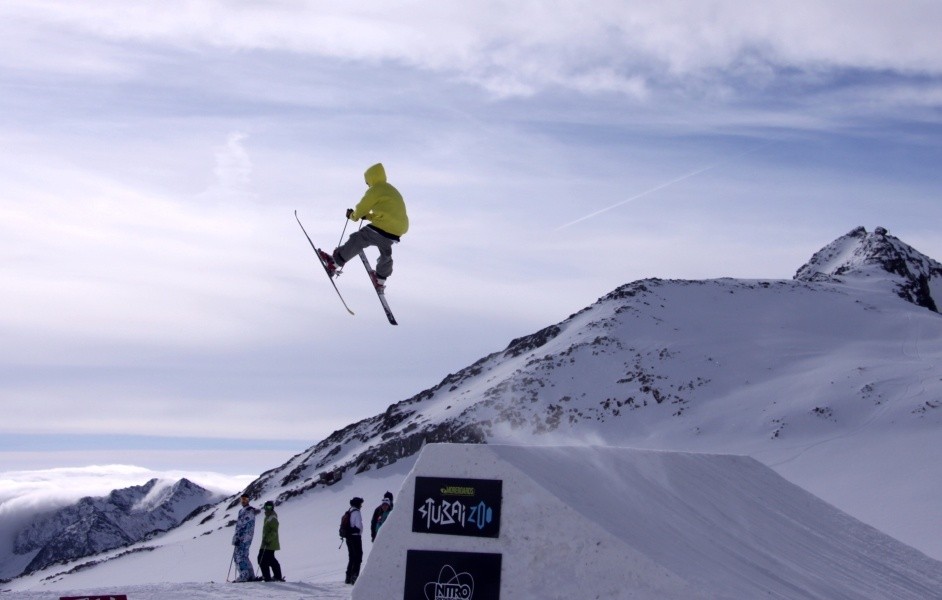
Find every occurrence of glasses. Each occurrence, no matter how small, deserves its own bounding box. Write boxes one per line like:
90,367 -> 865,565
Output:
265,506 -> 272,509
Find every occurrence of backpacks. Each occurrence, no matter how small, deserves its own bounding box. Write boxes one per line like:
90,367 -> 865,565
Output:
376,512 -> 390,532
339,509 -> 357,538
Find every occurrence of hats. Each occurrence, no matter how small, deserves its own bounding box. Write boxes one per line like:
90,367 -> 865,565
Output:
241,492 -> 249,498
350,497 -> 363,507
384,491 -> 393,501
264,501 -> 274,508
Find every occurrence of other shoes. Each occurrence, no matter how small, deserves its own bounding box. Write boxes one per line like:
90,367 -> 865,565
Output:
259,575 -> 268,581
272,576 -> 279,581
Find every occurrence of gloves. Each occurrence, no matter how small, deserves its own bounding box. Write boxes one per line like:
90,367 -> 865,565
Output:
346,208 -> 354,219
361,215 -> 366,221
234,537 -> 240,546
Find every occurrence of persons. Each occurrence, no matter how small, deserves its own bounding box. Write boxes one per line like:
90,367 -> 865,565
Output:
375,498 -> 392,534
370,489 -> 394,543
256,501 -> 286,582
230,493 -> 256,583
317,163 -> 410,295
337,496 -> 365,585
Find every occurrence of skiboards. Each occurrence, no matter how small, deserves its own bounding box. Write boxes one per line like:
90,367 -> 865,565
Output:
294,210 -> 398,325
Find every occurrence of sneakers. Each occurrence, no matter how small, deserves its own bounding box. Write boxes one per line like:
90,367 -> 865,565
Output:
319,250 -> 339,272
371,270 -> 386,286
